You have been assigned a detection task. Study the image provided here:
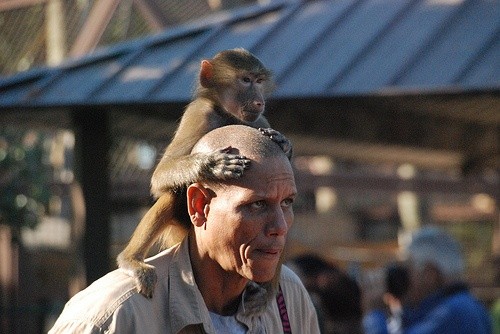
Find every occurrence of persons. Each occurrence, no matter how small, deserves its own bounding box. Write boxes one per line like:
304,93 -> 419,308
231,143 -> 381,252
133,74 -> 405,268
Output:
361,225 -> 492,334
46,124 -> 322,334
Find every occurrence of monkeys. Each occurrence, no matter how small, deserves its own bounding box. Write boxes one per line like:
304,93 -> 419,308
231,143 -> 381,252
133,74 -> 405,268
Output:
115,45 -> 293,319
